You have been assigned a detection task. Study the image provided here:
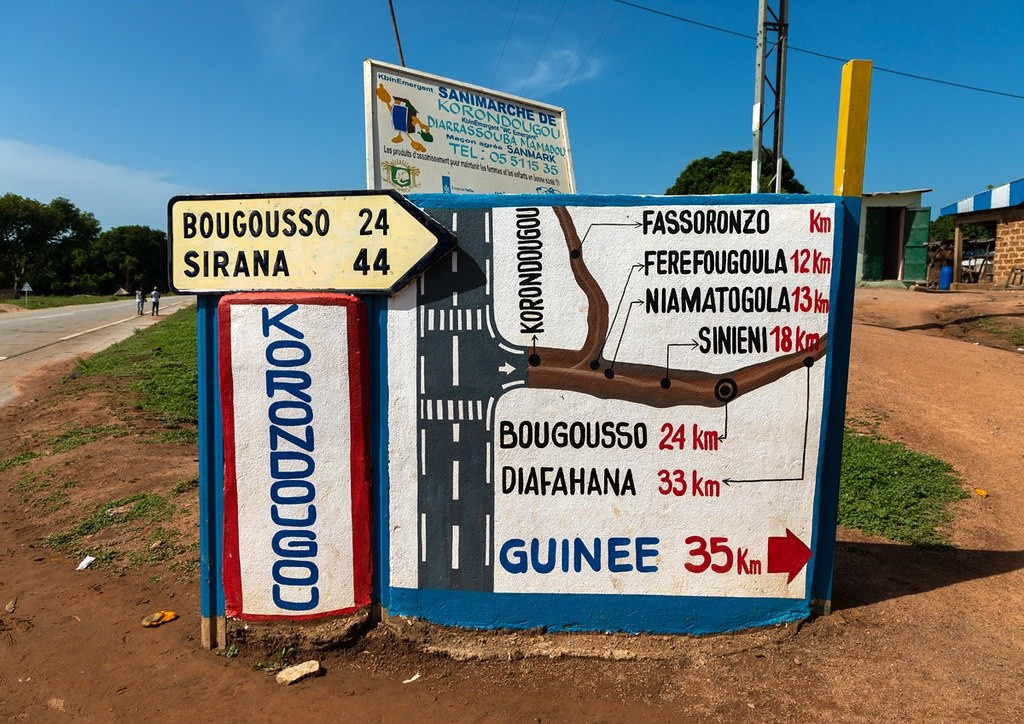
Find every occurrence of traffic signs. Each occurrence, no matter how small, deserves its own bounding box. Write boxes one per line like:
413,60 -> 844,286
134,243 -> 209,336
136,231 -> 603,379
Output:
166,192 -> 461,297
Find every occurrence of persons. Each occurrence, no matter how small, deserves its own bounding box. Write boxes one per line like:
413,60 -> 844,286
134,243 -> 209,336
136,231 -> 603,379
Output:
151,286 -> 161,316
135,285 -> 146,315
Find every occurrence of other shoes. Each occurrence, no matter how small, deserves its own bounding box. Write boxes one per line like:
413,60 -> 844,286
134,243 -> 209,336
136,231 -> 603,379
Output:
156,314 -> 158,316
152,314 -> 154,316
137,311 -> 139,315
141,311 -> 143,315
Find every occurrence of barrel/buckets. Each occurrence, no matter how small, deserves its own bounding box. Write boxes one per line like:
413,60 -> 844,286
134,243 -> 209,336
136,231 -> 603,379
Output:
939,267 -> 953,289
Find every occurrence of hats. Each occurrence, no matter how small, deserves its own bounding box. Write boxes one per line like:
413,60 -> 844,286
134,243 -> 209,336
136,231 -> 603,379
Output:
154,287 -> 157,290
